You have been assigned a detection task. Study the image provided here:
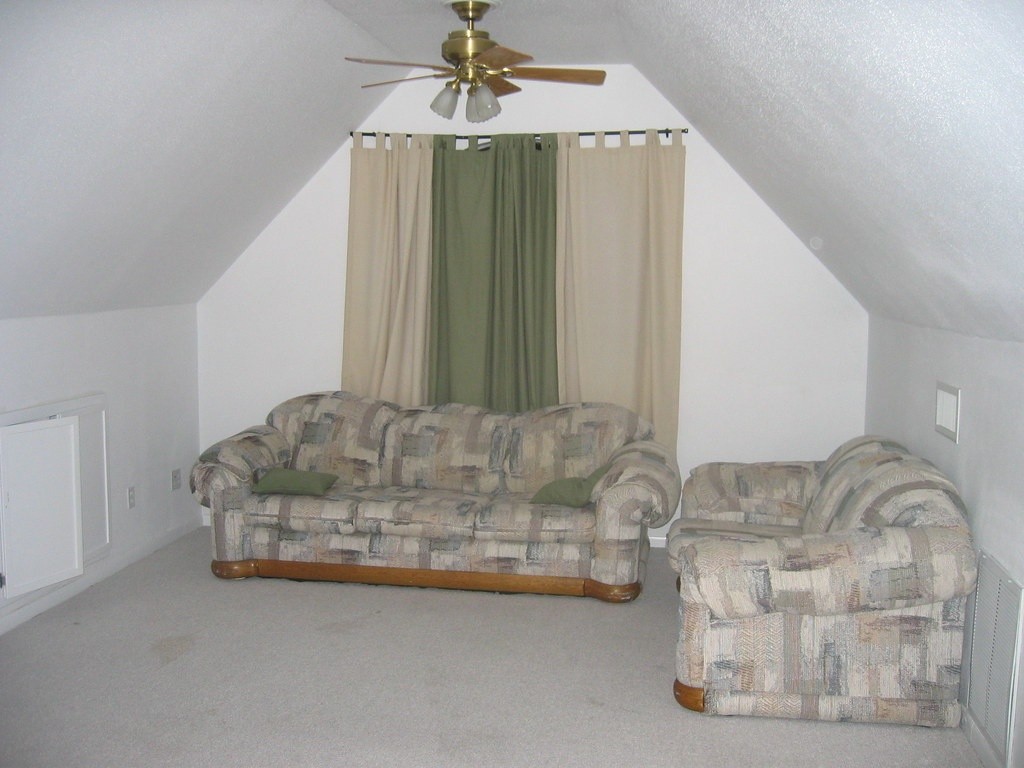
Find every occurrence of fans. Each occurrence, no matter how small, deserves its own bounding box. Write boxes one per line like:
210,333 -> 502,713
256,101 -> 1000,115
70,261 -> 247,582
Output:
345,0 -> 607,96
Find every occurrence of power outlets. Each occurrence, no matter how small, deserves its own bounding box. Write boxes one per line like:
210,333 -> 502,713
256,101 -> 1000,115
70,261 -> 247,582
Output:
172,469 -> 182,490
128,487 -> 135,508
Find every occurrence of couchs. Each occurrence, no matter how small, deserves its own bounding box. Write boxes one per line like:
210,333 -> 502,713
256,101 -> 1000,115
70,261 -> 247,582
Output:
667,433 -> 980,729
191,390 -> 681,603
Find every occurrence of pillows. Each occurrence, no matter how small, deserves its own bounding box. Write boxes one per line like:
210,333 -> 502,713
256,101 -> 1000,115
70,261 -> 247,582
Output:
250,466 -> 338,495
529,465 -> 614,506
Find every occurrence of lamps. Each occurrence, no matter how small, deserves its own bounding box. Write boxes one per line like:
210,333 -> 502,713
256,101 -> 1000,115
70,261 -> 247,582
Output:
430,77 -> 503,124
